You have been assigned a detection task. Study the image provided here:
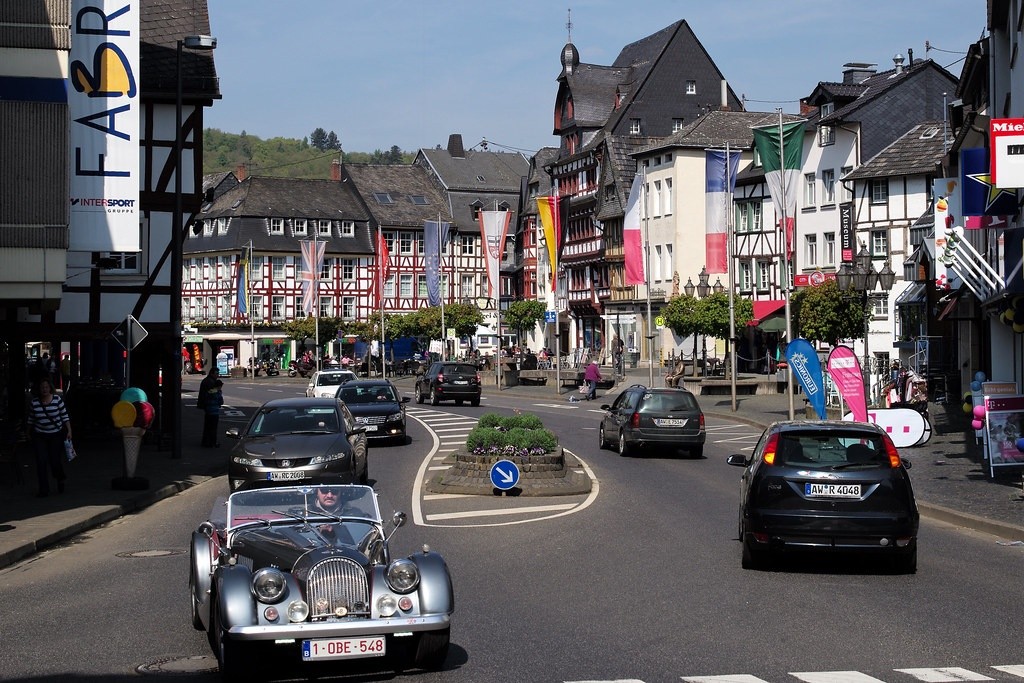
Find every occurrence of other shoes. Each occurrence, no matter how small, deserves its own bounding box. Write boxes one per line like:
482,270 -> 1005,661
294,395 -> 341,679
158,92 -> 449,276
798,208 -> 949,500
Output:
585,394 -> 590,401
38,487 -> 49,497
56,481 -> 65,494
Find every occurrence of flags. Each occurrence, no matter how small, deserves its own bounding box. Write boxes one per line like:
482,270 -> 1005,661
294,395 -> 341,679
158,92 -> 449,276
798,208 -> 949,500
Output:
704,149 -> 742,274
536,194 -> 571,292
238,246 -> 251,313
751,120 -> 806,261
623,173 -> 646,286
374,230 -> 389,310
478,210 -> 512,299
423,220 -> 450,306
301,240 -> 326,313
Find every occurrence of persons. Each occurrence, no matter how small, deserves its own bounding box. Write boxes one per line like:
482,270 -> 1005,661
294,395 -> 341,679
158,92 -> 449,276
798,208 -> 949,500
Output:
883,368 -> 918,408
301,350 -> 416,376
585,360 -> 602,401
612,335 -> 624,368
665,357 -> 685,388
24,351 -> 70,394
424,345 -> 554,384
197,367 -> 224,448
25,379 -> 72,495
375,388 -> 388,400
289,472 -> 372,518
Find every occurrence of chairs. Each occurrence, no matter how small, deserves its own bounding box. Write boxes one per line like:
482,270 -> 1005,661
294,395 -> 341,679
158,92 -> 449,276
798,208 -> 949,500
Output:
537,356 -> 570,370
846,444 -> 867,462
232,515 -> 284,527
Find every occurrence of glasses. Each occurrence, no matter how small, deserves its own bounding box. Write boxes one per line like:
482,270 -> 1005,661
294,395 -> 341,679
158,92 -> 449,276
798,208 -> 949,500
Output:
320,488 -> 339,494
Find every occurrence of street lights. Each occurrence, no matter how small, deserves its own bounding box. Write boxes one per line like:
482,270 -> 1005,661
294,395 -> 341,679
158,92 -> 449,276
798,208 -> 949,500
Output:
683,265 -> 724,378
836,241 -> 895,406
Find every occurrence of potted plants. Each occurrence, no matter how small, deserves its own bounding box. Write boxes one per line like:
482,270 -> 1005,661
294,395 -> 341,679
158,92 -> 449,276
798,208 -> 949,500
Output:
231,366 -> 247,378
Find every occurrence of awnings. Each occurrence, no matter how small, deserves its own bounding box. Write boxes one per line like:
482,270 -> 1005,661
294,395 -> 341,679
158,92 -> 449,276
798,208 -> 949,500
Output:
745,300 -> 786,332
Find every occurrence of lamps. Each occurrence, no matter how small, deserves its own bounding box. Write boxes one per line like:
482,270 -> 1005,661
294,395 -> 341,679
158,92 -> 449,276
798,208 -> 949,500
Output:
203,187 -> 215,202
192,219 -> 204,236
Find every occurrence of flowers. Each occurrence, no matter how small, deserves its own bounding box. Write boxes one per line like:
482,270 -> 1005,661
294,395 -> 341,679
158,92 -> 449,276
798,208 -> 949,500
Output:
466,412 -> 558,456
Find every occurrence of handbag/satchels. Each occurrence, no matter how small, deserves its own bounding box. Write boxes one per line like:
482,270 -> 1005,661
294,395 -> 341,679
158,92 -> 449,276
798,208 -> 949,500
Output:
64,437 -> 78,461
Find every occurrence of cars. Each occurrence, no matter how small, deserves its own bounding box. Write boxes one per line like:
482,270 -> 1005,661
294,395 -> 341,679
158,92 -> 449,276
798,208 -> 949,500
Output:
226,397 -> 367,495
189,484 -> 455,678
306,369 -> 359,398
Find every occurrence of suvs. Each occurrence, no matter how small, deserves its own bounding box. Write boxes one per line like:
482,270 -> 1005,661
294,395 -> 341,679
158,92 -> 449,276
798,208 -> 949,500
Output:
414,360 -> 481,405
336,378 -> 412,445
599,384 -> 706,456
727,420 -> 920,574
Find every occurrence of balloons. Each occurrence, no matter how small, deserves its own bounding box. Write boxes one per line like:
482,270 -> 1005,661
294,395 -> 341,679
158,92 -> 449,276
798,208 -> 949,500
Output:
1015,438 -> 1024,453
963,371 -> 986,430
999,295 -> 1024,334
